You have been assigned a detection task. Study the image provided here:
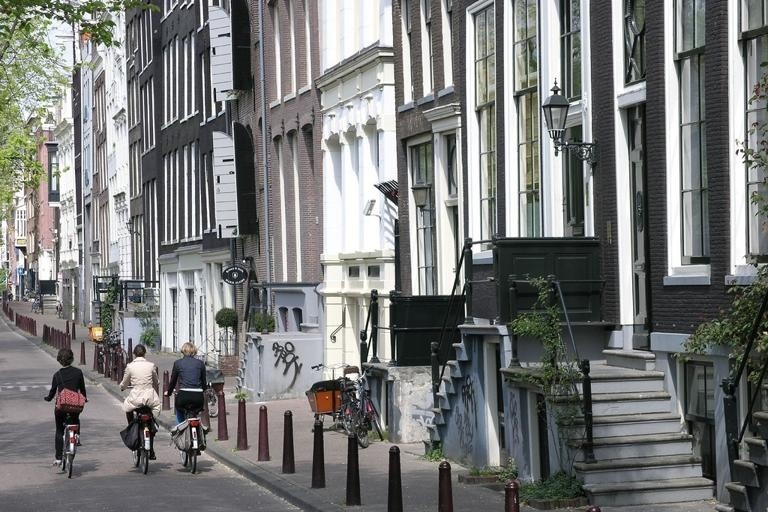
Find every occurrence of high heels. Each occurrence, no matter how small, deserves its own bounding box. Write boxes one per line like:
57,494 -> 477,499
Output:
74,433 -> 80,444
53,459 -> 62,469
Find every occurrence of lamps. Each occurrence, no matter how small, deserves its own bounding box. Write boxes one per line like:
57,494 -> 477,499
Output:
330,305 -> 346,343
542,78 -> 596,169
411,179 -> 431,214
126,219 -> 141,236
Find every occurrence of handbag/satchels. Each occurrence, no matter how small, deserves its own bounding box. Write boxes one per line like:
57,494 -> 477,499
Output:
119,419 -> 142,450
171,420 -> 192,452
198,419 -> 209,451
56,388 -> 86,412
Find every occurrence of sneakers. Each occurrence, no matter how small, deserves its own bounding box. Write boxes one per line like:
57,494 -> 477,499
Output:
149,450 -> 156,460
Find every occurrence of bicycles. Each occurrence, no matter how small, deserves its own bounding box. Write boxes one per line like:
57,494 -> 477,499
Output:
44,395 -> 89,478
165,389 -> 203,474
311,362 -> 384,448
120,386 -> 156,475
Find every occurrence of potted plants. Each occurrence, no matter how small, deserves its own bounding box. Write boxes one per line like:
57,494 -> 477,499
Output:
216,308 -> 238,376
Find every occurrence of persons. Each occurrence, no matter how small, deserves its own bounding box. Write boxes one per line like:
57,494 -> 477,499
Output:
8,293 -> 14,301
121,344 -> 161,460
163,342 -> 207,424
44,346 -> 89,467
